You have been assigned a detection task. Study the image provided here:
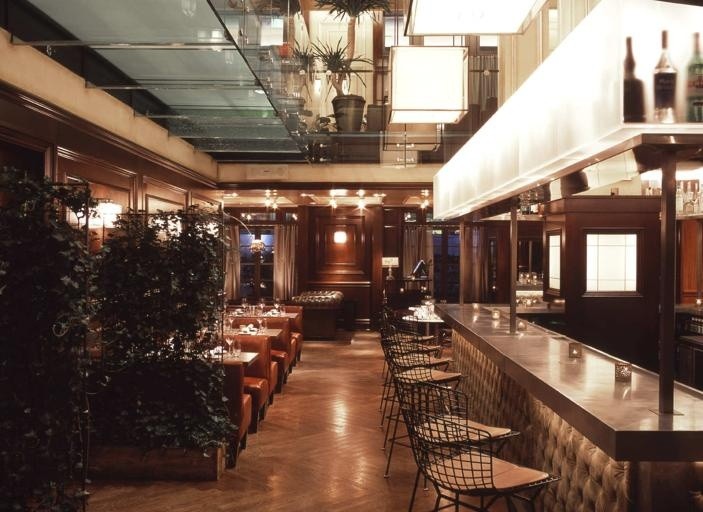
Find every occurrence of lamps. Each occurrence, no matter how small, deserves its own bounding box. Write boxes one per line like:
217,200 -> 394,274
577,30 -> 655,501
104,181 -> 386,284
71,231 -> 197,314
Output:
382,257 -> 399,279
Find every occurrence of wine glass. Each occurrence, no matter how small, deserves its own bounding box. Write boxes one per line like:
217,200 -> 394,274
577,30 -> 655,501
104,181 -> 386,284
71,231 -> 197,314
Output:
211,337 -> 242,360
259,319 -> 268,336
227,311 -> 235,333
408,299 -> 435,321
226,297 -> 286,317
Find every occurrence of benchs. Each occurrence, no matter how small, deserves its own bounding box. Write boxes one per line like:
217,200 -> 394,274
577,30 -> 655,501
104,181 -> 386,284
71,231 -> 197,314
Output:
203,299 -> 306,466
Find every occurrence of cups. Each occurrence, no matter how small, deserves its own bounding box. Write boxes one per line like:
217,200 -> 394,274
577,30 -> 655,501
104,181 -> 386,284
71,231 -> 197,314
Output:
239,325 -> 246,331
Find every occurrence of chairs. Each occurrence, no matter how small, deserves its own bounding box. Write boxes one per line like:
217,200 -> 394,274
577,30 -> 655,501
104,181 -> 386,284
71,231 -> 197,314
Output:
377,304 -> 553,511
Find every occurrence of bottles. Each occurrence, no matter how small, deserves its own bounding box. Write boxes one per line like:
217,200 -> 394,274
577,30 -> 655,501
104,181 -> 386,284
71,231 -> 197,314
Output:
653,31 -> 678,122
623,35 -> 646,123
687,30 -> 703,122
676,180 -> 702,213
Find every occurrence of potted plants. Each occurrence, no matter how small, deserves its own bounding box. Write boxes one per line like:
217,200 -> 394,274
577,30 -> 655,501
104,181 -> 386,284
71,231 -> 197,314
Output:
310,0 -> 391,132
